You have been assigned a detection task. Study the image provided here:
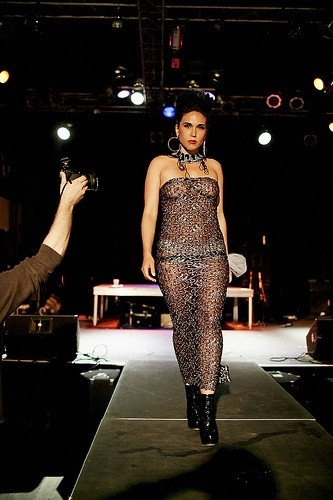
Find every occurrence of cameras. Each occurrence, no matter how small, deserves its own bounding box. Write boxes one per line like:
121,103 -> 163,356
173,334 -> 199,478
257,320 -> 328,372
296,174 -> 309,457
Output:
60,157 -> 99,192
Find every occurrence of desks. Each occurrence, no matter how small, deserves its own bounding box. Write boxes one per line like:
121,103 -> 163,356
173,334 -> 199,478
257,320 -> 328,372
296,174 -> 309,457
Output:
93,284 -> 253,330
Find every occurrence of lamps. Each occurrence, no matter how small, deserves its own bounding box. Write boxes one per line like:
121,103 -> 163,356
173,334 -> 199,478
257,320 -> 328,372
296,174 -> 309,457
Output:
0,0 -> 333,145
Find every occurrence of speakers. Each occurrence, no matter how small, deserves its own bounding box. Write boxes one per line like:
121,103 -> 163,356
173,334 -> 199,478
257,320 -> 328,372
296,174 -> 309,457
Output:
304,319 -> 333,363
6,315 -> 80,361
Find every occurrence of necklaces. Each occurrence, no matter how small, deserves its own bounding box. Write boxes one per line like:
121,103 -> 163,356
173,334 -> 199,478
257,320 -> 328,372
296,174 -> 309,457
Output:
169,150 -> 209,178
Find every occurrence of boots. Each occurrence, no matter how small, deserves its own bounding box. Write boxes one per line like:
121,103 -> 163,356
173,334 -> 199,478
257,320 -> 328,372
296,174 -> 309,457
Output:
185,384 -> 199,430
197,392 -> 219,446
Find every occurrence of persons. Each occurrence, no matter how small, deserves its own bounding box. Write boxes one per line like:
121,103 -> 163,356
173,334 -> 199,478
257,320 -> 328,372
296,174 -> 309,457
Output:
140,90 -> 247,447
0,171 -> 88,325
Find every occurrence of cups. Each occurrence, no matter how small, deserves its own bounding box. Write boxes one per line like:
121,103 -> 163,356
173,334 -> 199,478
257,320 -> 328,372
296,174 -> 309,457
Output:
113,279 -> 119,285
